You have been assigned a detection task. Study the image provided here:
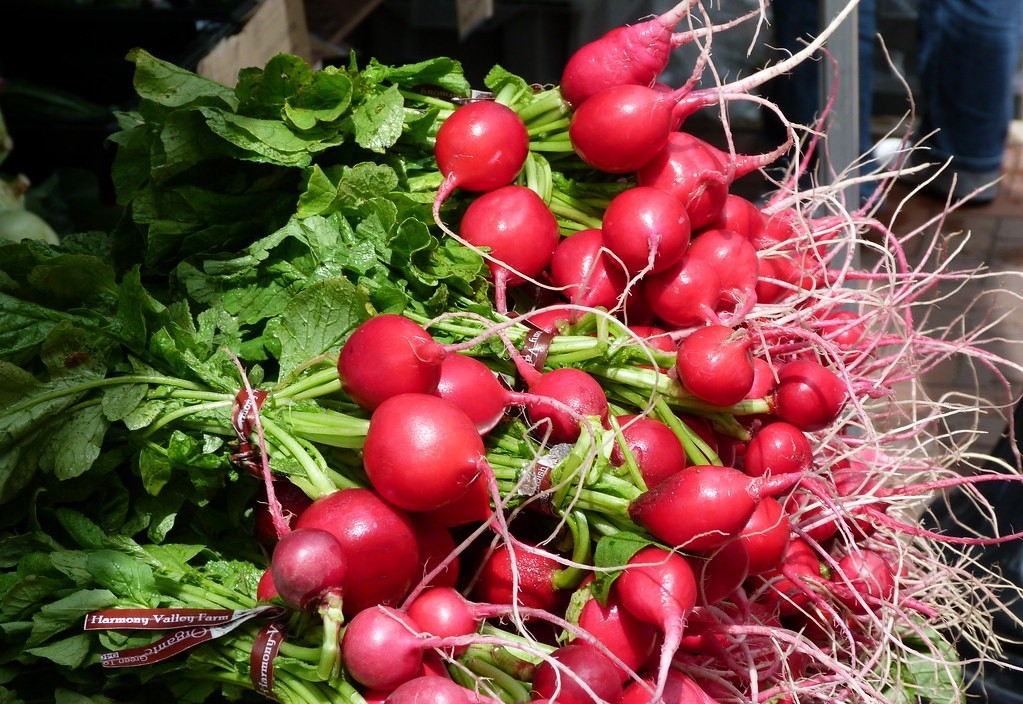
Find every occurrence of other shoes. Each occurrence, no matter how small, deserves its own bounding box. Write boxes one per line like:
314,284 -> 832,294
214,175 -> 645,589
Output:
873,138 -> 926,188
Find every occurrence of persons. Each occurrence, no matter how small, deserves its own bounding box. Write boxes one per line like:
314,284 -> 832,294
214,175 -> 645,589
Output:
753,0 -> 1023,222
302,0 -> 654,109
